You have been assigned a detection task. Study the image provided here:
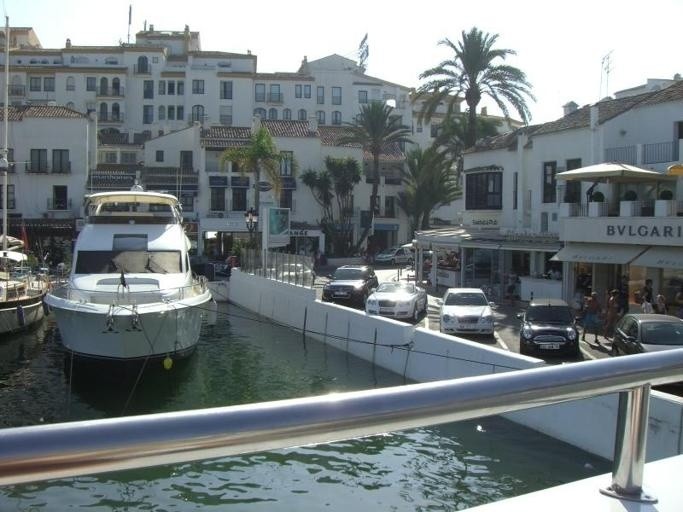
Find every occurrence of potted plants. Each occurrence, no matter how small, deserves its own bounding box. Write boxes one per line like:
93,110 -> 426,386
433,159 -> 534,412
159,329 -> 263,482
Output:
561,190 -> 676,216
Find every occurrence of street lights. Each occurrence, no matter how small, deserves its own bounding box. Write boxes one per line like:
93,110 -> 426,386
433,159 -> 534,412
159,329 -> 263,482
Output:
244,206 -> 259,250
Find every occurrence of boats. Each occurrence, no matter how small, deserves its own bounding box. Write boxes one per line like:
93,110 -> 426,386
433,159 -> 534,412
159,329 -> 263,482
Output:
46,179 -> 214,361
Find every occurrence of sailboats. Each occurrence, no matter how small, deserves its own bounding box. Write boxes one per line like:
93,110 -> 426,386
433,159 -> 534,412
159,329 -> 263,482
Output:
0,16 -> 45,362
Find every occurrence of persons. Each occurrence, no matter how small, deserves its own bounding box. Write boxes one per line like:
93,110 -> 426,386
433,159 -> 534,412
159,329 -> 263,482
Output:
582,279 -> 683,343
506,268 -> 518,303
547,266 -> 562,280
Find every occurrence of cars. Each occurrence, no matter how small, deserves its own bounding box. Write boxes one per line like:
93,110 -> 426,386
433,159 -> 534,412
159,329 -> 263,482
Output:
321,242 -> 433,320
435,287 -> 495,337
612,314 -> 683,358
517,297 -> 583,355
